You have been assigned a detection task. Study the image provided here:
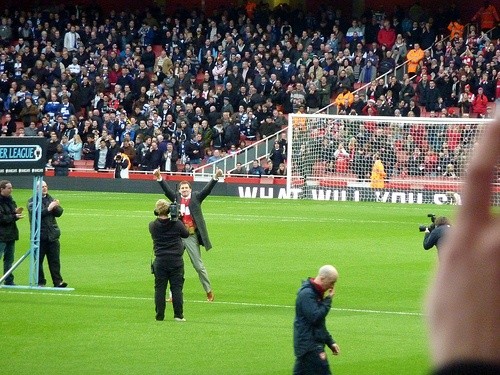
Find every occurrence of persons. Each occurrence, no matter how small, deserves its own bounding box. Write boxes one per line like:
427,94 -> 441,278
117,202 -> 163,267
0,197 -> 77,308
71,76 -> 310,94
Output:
421,109 -> 500,375
26,180 -> 68,288
0,180 -> 26,287
0,0 -> 500,205
292,264 -> 341,375
423,216 -> 455,261
152,169 -> 223,302
149,199 -> 190,321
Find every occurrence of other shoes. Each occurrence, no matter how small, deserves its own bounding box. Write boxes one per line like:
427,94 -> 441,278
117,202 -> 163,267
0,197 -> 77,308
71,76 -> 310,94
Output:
39,278 -> 47,286
207,292 -> 214,302
175,316 -> 186,322
4,281 -> 16,286
54,280 -> 67,287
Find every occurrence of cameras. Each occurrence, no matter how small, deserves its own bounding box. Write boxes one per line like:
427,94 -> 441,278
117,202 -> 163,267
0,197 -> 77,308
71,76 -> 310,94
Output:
419,213 -> 437,232
